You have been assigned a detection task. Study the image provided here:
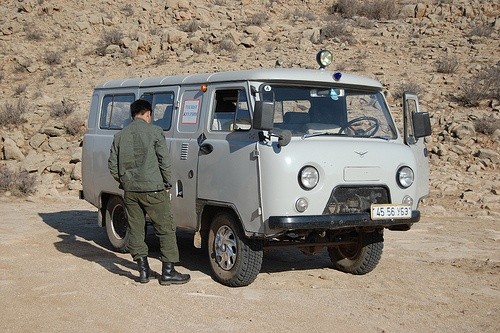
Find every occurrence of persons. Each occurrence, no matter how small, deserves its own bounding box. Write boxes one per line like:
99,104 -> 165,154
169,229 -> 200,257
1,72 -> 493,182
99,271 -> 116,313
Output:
107,99 -> 191,286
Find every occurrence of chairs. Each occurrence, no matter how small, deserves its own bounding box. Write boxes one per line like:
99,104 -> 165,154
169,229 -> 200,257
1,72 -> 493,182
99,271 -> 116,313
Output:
157,106 -> 172,128
284,112 -> 311,123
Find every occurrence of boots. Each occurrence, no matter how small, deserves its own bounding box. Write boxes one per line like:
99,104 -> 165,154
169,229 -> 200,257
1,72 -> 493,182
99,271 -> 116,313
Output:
159,262 -> 190,284
136,256 -> 161,283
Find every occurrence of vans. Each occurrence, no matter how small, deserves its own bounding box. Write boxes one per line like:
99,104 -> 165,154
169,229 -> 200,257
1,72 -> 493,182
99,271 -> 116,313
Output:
79,49 -> 433,288
